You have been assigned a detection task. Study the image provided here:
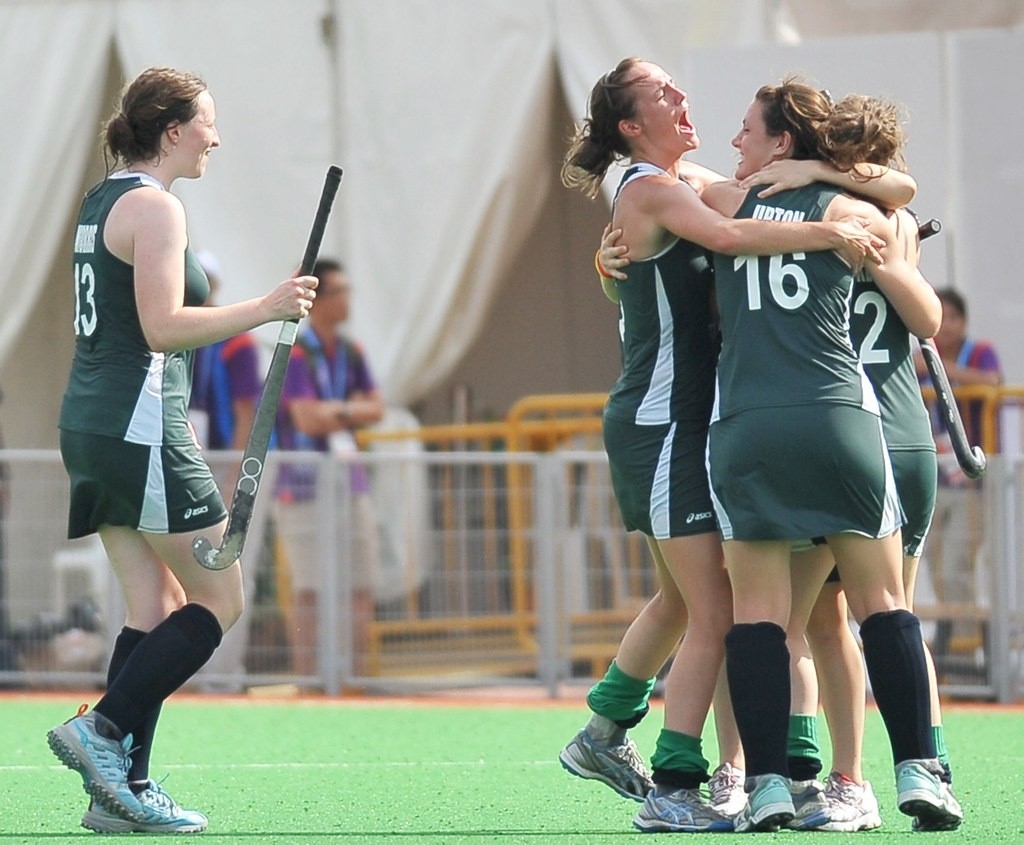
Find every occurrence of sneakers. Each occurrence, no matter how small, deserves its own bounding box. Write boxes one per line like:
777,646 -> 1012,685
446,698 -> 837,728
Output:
911,789 -> 961,832
733,773 -> 797,832
46,703 -> 146,824
632,787 -> 734,832
780,779 -> 832,831
707,762 -> 750,817
895,763 -> 964,825
558,729 -> 657,803
812,772 -> 882,833
79,772 -> 208,835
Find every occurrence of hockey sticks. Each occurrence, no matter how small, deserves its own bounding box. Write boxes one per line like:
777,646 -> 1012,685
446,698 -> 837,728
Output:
916,218 -> 943,240
919,333 -> 988,481
190,165 -> 343,569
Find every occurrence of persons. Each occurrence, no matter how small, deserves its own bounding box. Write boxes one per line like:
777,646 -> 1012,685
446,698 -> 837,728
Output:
45,67 -> 320,834
910,290 -> 1014,672
185,251 -> 261,696
269,260 -> 383,700
560,55 -> 965,833
